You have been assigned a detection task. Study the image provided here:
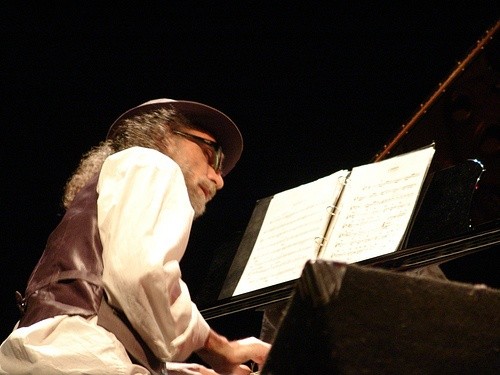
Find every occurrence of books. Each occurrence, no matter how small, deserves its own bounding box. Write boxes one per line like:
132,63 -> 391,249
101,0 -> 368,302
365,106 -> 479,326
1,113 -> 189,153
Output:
233,147 -> 435,296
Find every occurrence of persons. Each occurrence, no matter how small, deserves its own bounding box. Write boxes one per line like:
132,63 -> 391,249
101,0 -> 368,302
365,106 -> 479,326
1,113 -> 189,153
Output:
0,97 -> 272,375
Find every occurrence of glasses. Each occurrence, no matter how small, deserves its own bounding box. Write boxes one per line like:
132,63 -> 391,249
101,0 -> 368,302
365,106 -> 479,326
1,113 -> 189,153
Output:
175,131 -> 225,171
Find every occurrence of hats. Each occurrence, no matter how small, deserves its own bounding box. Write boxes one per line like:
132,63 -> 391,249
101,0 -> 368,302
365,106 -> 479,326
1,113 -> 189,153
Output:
106,99 -> 243,177
